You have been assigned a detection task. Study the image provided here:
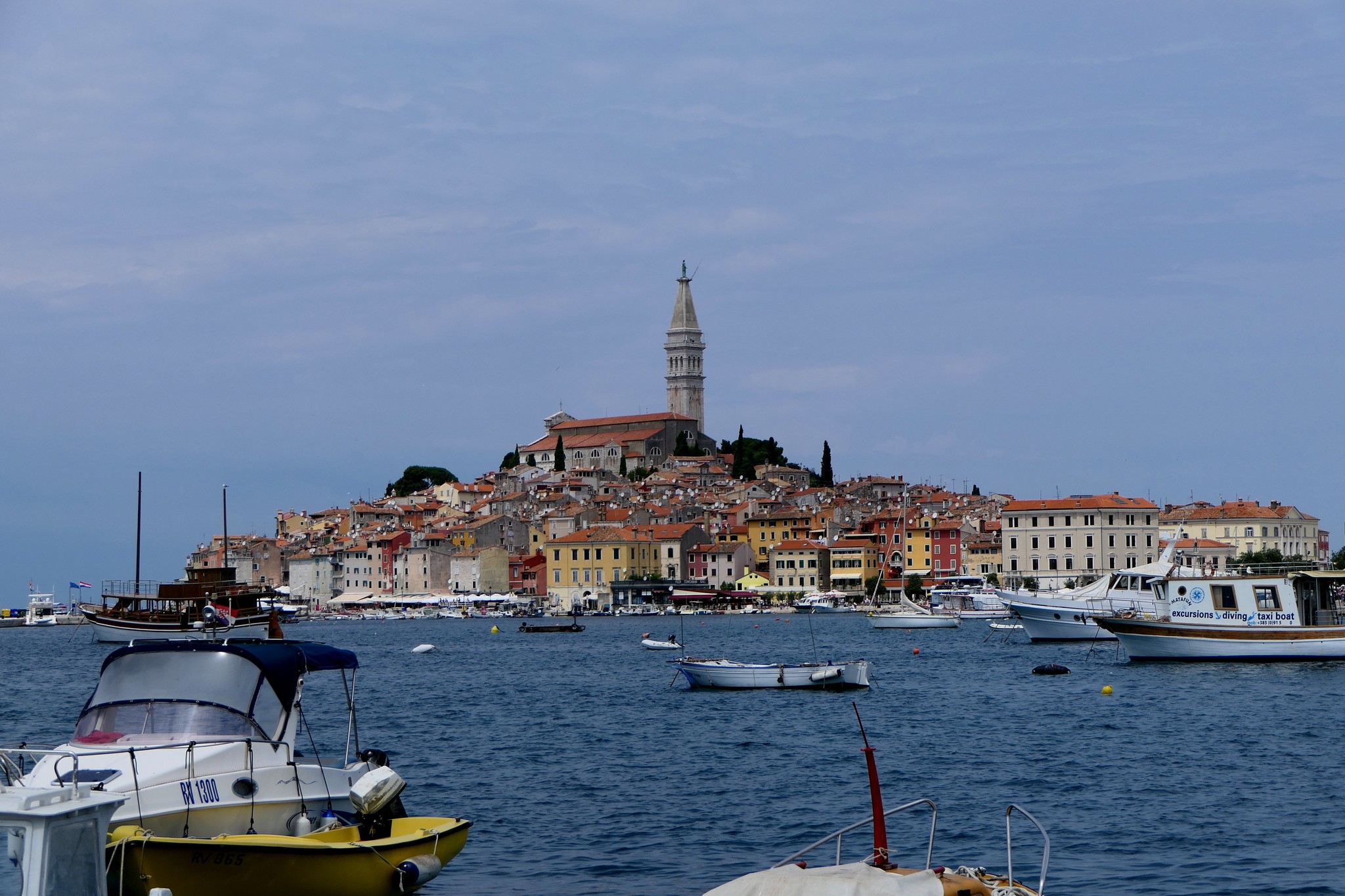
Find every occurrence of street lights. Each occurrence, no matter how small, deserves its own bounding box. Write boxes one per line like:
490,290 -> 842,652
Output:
489,586 -> 492,602
801,584 -> 804,597
932,579 -> 935,586
401,586 -> 404,607
309,587 -> 312,613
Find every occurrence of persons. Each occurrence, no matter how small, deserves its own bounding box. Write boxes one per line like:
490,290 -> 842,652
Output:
518,612 -> 524,617
936,581 -> 965,587
1340,584 -> 1345,607
360,606 -> 363,612
466,598 -> 470,604
715,596 -> 768,607
439,597 -> 461,605
778,597 -> 875,610
373,604 -> 386,609
678,599 -> 711,608
926,599 -> 929,604
321,603 -> 347,613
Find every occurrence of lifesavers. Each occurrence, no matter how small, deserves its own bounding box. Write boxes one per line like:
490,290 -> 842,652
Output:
571,624 -> 578,631
583,627 -> 585,630
530,625 -> 534,626
1166,564 -> 1177,577
1202,561 -> 1215,577
519,626 -> 525,632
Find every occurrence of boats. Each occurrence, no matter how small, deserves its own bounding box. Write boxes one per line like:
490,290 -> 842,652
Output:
76,471 -> 285,646
989,504 -> 1247,643
641,639 -> 678,650
0,636 -> 474,896
1084,519 -> 1345,662
256,597 -> 308,623
0,577 -> 91,628
308,574 -> 1015,621
664,614 -> 874,689
701,701 -> 1053,896
989,623 -> 1026,631
518,614 -> 586,633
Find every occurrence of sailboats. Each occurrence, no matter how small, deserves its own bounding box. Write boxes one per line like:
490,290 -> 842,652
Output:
865,483 -> 961,628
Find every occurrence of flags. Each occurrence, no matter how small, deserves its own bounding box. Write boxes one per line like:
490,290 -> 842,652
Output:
206,600 -> 238,628
65,603 -> 69,606
30,589 -> 35,592
37,591 -> 40,593
29,582 -> 32,585
80,581 -> 91,588
29,586 -> 34,588
70,582 -> 79,589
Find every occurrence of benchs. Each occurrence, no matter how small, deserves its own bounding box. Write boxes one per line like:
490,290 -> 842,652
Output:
249,589 -> 258,593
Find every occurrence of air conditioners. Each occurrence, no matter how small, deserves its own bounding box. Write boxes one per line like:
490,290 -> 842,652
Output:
596,582 -> 600,585
578,582 -> 583,585
887,561 -> 890,564
602,582 -> 606,585
548,592 -> 553,595
888,570 -> 892,572
